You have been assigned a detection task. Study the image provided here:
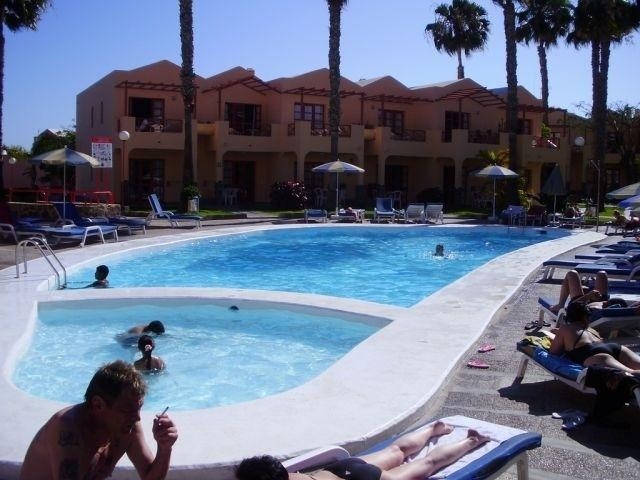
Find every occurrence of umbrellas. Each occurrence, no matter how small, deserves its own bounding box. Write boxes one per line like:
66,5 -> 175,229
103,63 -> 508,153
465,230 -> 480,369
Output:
474,162 -> 520,217
619,195 -> 640,208
543,161 -> 567,224
312,157 -> 367,215
605,182 -> 640,200
30,145 -> 106,225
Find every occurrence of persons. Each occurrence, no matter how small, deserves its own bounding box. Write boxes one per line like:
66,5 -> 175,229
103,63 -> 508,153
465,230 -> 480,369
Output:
232,421 -> 492,479
93,264 -> 109,288
128,320 -> 165,336
549,269 -> 628,312
565,206 -> 582,217
134,334 -> 165,372
434,244 -> 444,256
347,207 -> 360,220
18,360 -> 180,480
139,117 -> 154,132
611,209 -> 640,230
548,299 -> 640,376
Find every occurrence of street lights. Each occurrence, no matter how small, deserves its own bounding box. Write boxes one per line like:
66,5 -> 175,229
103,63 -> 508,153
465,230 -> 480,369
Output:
574,135 -> 586,194
120,129 -> 130,210
8,158 -> 17,201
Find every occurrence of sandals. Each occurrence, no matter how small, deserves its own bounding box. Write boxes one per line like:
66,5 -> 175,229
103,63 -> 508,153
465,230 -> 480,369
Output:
551,408 -> 589,419
562,416 -> 585,430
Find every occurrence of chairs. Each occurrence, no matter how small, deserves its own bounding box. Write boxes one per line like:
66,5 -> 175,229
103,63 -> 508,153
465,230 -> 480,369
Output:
0,193 -> 205,247
304,197 -> 444,223
280,416 -> 541,480
499,204 -> 640,408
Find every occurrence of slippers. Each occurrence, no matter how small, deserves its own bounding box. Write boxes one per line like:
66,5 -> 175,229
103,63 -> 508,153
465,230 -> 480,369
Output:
477,343 -> 496,352
467,359 -> 491,368
533,319 -> 552,327
525,319 -> 540,330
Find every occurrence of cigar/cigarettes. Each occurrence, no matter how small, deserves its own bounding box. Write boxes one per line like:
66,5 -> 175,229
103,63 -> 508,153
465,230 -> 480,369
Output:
158,406 -> 169,421
